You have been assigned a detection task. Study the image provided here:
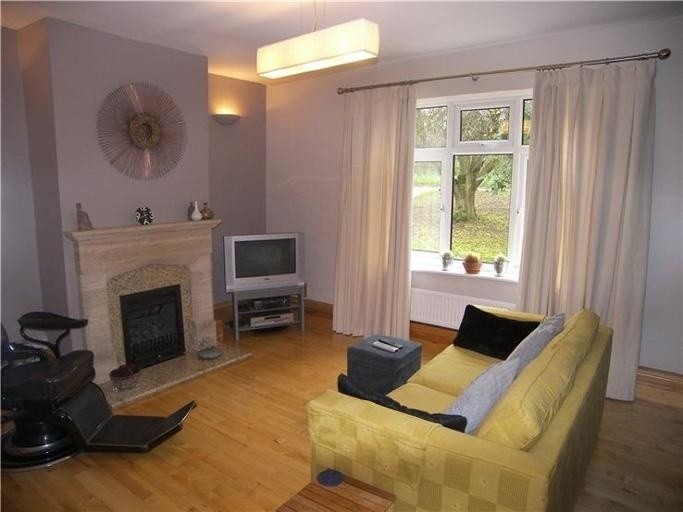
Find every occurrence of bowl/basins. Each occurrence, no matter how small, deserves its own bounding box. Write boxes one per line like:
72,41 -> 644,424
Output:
109,369 -> 142,390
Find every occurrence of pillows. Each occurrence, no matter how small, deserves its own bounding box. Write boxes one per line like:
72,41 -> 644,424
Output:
509,313 -> 565,371
453,303 -> 540,360
336,374 -> 467,440
447,359 -> 521,439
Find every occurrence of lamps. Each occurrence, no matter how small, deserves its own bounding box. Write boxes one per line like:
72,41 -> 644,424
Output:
211,112 -> 244,127
255,0 -> 381,81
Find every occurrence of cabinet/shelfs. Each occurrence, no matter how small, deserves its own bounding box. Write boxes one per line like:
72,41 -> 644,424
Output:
231,282 -> 307,341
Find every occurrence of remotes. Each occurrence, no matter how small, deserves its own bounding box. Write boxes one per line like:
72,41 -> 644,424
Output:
372,341 -> 399,352
378,337 -> 404,349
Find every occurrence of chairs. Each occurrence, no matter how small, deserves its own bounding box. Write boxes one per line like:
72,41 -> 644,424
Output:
0,311 -> 196,473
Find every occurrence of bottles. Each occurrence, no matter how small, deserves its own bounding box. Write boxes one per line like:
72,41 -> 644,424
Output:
187,201 -> 214,221
76,203 -> 86,231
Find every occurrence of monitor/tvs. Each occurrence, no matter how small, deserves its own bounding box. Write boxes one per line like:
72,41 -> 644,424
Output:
223,231 -> 306,293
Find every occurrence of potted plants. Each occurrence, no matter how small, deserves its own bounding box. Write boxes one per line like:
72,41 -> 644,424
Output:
463,252 -> 482,275
439,250 -> 455,271
494,255 -> 506,277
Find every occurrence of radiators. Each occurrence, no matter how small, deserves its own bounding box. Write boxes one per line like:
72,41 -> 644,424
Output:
410,287 -> 518,332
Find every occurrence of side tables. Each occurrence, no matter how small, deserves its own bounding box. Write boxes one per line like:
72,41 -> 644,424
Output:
275,469 -> 396,512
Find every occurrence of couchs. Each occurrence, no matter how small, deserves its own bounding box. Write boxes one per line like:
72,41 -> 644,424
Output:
309,303 -> 613,512
